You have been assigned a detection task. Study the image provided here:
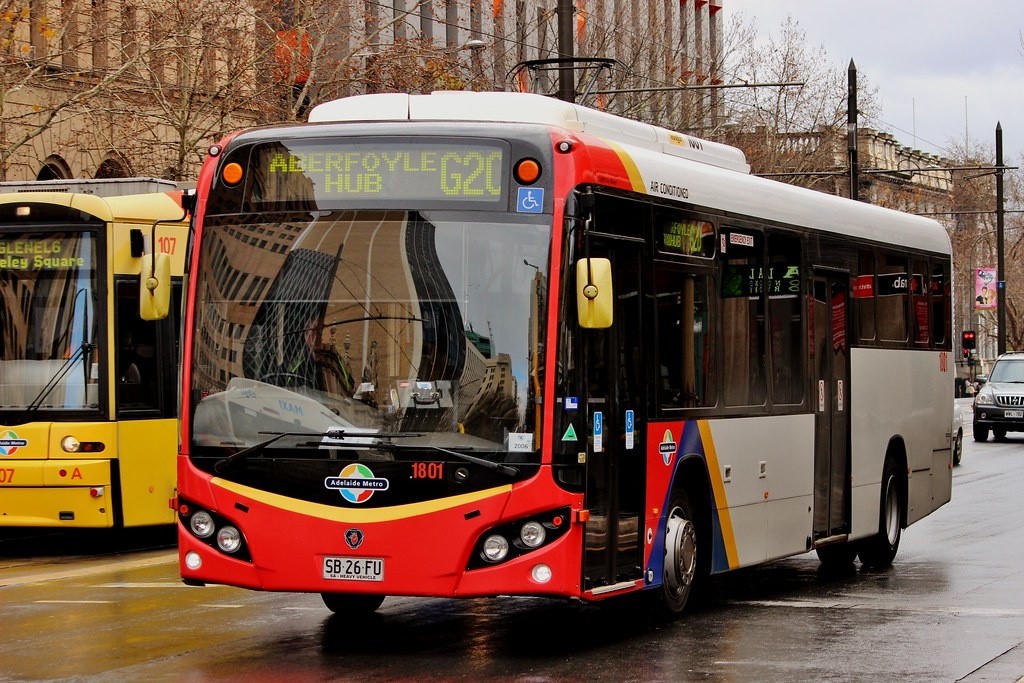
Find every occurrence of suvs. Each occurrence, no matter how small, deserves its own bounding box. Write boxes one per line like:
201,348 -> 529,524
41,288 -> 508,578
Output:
969,350 -> 1024,441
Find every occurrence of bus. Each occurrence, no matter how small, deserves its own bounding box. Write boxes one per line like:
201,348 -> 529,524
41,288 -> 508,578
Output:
138,90 -> 957,623
0,179 -> 216,532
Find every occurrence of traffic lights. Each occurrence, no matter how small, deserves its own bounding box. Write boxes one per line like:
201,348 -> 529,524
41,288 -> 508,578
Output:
961,330 -> 975,350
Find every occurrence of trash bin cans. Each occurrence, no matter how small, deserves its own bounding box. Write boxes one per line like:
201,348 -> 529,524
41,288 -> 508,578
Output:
955,377 -> 974,398
